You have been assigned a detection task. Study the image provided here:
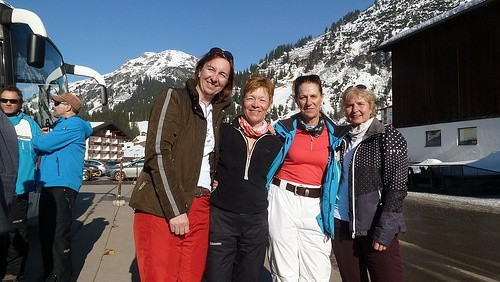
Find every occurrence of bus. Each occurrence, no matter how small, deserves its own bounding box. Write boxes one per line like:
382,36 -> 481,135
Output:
0,1 -> 110,194
367,1 -> 500,193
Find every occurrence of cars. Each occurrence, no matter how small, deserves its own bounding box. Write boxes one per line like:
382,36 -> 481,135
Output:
82,154 -> 145,182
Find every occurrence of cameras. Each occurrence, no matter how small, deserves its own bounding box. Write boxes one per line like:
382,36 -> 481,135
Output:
43,119 -> 52,133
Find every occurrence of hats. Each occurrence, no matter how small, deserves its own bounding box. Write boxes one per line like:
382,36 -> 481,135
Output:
51,93 -> 82,112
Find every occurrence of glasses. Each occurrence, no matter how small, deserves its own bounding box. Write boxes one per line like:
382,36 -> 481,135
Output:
208,47 -> 234,69
295,75 -> 321,82
345,84 -> 367,91
53,101 -> 69,106
0,98 -> 20,104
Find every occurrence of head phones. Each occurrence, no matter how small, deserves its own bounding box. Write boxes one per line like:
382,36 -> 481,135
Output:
298,115 -> 326,134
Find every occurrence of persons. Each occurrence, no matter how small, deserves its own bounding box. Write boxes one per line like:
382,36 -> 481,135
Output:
129,48 -> 277,282
213,75 -> 342,282
0,86 -> 43,282
0,110 -> 18,282
209,75 -> 283,282
31,92 -> 93,282
331,87 -> 409,282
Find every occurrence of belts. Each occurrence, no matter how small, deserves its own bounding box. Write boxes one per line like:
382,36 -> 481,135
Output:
270,177 -> 321,198
194,187 -> 210,197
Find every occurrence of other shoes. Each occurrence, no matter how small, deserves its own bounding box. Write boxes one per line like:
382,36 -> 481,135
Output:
2,272 -> 19,281
